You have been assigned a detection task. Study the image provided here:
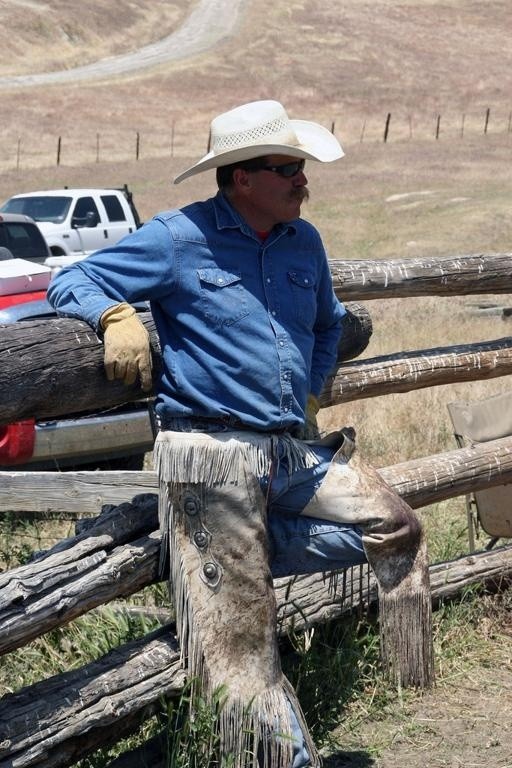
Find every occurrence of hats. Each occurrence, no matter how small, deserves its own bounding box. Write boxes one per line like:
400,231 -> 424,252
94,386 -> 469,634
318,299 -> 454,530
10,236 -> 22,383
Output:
173,100 -> 345,184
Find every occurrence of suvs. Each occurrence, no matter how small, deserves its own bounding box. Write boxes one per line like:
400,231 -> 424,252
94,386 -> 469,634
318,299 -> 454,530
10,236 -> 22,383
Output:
0,183 -> 142,262
0,212 -> 161,476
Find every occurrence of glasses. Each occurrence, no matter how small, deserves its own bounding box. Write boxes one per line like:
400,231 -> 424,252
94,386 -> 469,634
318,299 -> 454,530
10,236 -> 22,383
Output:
263,160 -> 306,178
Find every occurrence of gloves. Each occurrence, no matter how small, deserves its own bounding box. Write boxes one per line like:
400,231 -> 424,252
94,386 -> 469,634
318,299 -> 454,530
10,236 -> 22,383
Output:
100,301 -> 154,391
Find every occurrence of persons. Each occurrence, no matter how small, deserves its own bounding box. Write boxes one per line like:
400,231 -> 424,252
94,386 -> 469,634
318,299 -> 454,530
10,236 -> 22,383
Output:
44,98 -> 425,768
